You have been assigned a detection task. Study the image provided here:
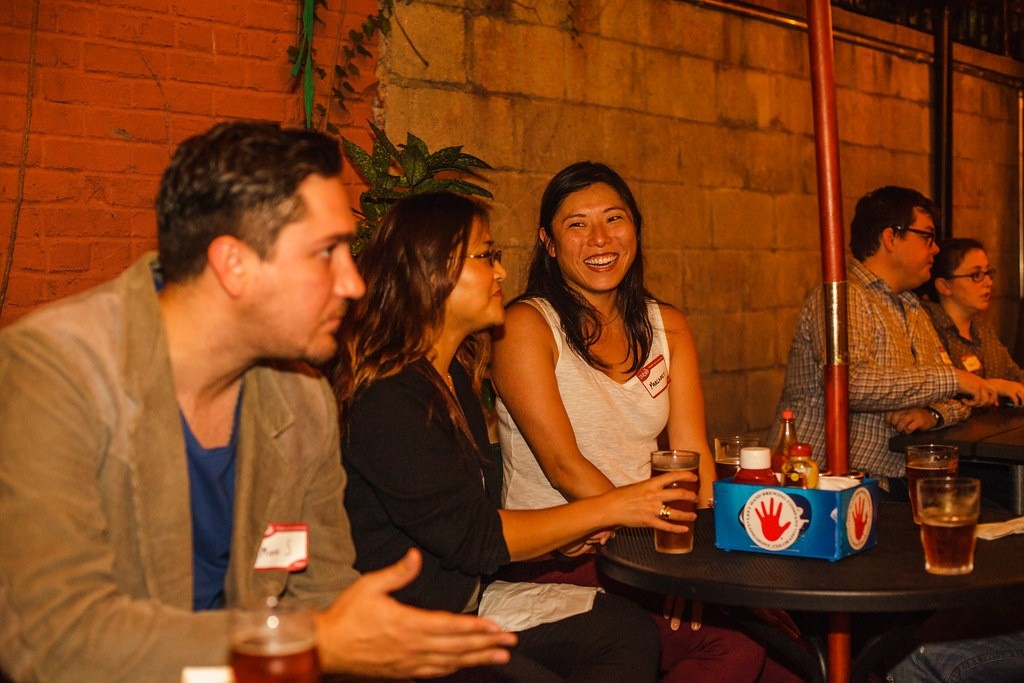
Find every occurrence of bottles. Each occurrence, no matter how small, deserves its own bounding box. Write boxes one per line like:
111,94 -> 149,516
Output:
733,446 -> 780,486
771,409 -> 798,472
780,443 -> 820,489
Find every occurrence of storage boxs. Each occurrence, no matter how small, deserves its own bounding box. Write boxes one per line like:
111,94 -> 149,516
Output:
713,475 -> 880,563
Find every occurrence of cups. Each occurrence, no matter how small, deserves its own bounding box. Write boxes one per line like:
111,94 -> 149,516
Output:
650,450 -> 700,554
904,444 -> 960,525
714,435 -> 761,480
916,476 -> 981,576
225,572 -> 322,683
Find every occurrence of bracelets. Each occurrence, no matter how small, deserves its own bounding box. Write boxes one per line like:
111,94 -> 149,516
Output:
926,407 -> 943,429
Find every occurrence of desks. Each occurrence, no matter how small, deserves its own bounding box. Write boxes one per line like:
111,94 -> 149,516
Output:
889,402 -> 1024,515
594,502 -> 1024,683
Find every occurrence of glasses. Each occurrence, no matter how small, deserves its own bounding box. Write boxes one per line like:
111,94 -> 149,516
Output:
944,269 -> 995,282
891,225 -> 936,246
447,249 -> 502,266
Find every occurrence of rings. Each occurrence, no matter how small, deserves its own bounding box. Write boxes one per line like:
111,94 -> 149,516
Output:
659,506 -> 671,519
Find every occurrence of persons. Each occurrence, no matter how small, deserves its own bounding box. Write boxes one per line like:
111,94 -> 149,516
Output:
1,119 -> 517,683
763,185 -> 1024,502
911,238 -> 1024,413
490,161 -> 820,683
884,625 -> 1023,683
322,191 -> 700,683
